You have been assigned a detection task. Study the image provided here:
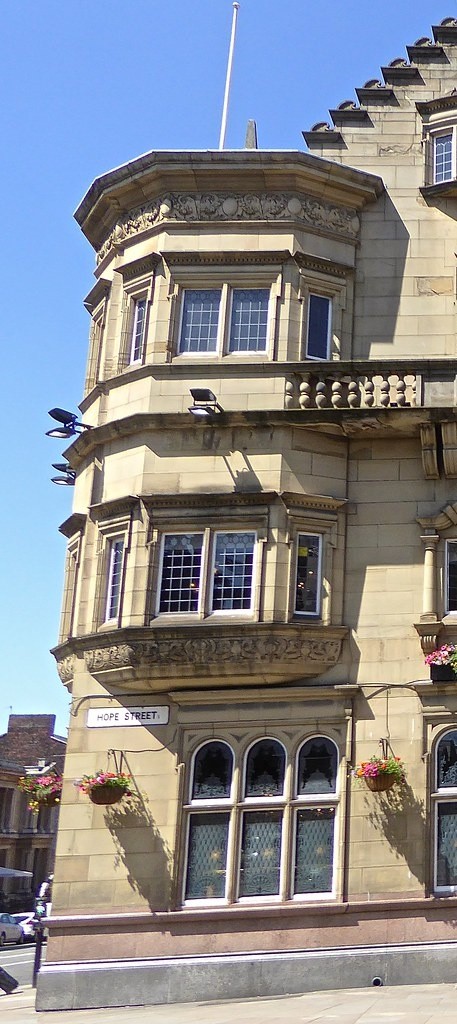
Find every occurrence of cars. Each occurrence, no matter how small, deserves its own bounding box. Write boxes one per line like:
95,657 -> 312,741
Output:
0,912 -> 25,947
11,912 -> 49,943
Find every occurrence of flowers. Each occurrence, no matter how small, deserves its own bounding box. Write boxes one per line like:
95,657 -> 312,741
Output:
424,641 -> 457,673
14,769 -> 62,816
72,769 -> 149,806
345,754 -> 409,788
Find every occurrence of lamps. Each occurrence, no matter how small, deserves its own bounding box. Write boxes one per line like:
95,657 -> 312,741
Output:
188,388 -> 223,417
44,408 -> 93,439
50,463 -> 75,486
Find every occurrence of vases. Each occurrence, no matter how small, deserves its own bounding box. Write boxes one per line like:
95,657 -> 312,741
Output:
86,786 -> 125,804
30,790 -> 61,807
429,664 -> 457,682
363,772 -> 397,792
